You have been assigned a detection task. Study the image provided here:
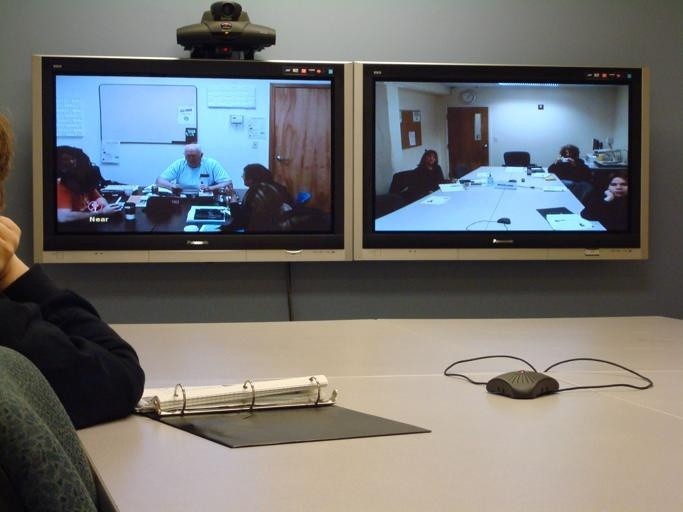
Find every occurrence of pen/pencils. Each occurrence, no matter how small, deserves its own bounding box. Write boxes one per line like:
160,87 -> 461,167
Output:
498,183 -> 513,186
115,197 -> 121,204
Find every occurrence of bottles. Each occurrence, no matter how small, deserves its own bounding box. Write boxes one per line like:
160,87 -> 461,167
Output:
487,171 -> 493,186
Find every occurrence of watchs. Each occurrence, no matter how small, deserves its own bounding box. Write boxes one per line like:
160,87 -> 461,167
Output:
547,145 -> 590,188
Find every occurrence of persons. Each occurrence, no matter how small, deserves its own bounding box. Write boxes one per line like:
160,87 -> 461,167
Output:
0,109 -> 145,431
55,146 -> 127,222
410,150 -> 456,199
228,163 -> 293,234
569,171 -> 629,247
155,143 -> 233,193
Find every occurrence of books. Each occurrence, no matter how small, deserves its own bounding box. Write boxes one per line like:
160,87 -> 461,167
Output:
185,203 -> 226,223
545,212 -> 593,231
438,181 -> 464,193
543,185 -> 564,192
101,183 -> 138,194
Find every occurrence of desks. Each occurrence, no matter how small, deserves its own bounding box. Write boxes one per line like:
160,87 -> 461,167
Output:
93,187 -> 249,232
374,164 -> 607,230
65,312 -> 682,512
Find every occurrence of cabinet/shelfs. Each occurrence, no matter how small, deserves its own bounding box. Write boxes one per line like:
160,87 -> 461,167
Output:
583,155 -> 626,190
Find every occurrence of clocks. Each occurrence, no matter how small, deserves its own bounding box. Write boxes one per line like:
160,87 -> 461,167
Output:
459,89 -> 476,106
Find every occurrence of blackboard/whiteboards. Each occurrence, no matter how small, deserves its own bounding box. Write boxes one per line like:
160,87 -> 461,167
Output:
99,84 -> 198,144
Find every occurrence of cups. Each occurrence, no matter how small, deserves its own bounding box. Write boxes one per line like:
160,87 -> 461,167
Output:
124,201 -> 135,221
464,181 -> 471,191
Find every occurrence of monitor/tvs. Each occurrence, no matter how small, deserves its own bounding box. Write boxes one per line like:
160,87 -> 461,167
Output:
353,59 -> 650,263
31,52 -> 352,263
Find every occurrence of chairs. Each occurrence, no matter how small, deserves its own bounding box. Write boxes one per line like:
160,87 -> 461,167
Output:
568,181 -> 594,204
501,150 -> 532,166
386,169 -> 424,211
374,192 -> 406,221
451,162 -> 474,178
293,190 -> 313,206
